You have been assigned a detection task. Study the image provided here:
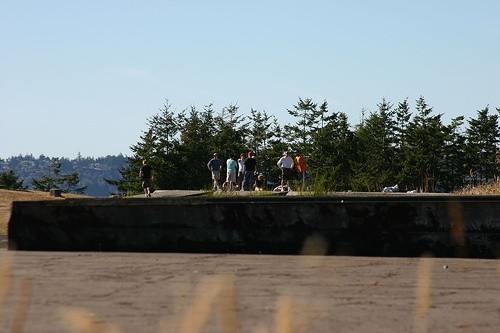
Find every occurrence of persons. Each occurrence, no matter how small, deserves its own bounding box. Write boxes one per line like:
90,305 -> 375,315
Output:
253,174 -> 264,191
278,150 -> 295,192
240,152 -> 257,191
470,164 -> 479,189
294,149 -> 307,190
138,160 -> 153,198
207,153 -> 223,191
238,154 -> 246,189
222,154 -> 238,191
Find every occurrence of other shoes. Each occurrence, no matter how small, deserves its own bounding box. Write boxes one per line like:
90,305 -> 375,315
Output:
145,194 -> 151,197
281,188 -> 284,192
288,187 -> 293,192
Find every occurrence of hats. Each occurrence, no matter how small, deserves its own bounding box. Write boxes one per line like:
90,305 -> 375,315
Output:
240,153 -> 246,156
143,160 -> 150,166
248,152 -> 252,157
282,151 -> 289,156
213,153 -> 218,156
